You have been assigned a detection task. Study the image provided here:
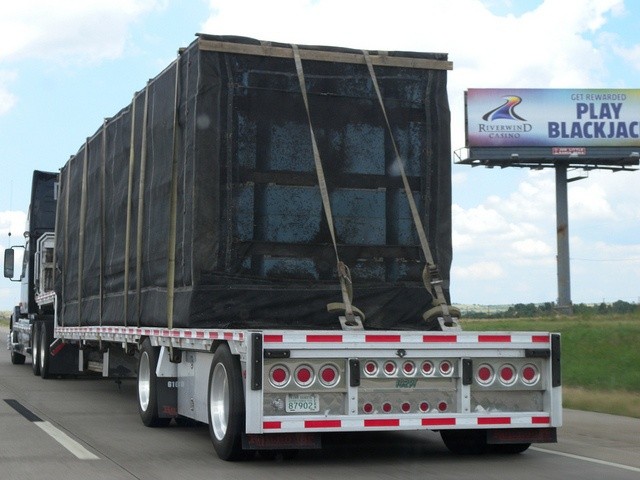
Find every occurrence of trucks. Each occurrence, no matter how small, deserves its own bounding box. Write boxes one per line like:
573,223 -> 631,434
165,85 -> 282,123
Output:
4,33 -> 563,460
